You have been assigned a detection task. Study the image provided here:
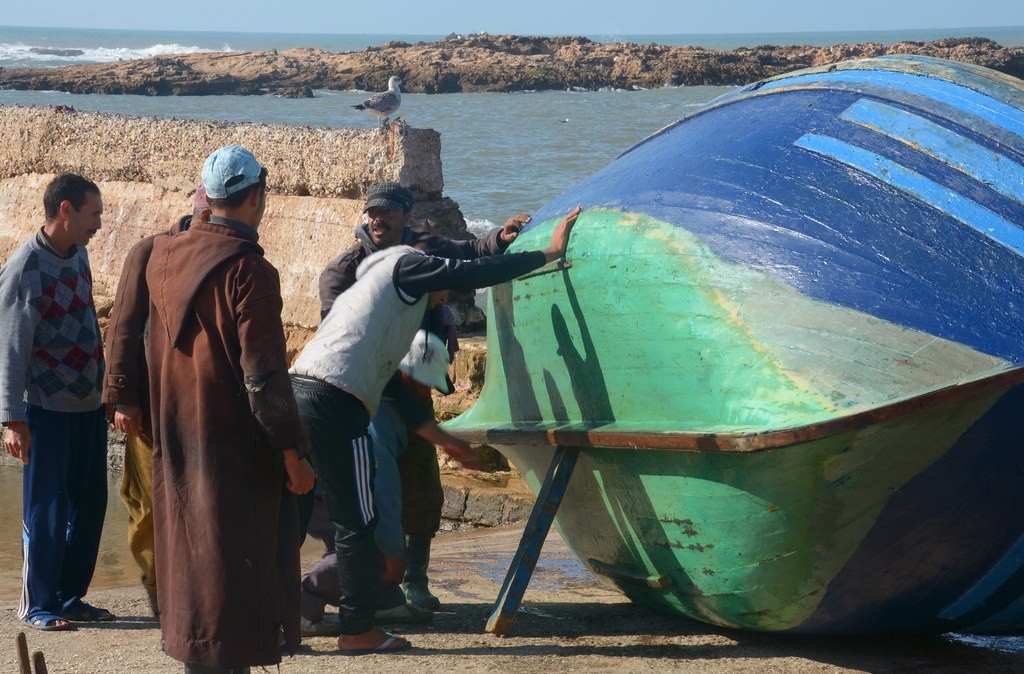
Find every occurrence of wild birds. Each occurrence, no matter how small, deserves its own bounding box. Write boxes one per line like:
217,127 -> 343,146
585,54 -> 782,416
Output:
558,119 -> 570,123
349,76 -> 407,130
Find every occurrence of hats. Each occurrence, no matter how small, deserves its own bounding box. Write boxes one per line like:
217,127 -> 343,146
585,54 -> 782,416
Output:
363,181 -> 415,214
397,327 -> 450,395
201,146 -> 261,199
194,183 -> 210,207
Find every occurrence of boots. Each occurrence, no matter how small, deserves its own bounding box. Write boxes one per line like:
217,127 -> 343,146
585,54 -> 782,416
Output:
402,532 -> 439,609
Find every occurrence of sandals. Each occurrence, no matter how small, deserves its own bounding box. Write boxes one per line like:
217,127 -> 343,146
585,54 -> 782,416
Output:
19,613 -> 71,631
78,604 -> 117,621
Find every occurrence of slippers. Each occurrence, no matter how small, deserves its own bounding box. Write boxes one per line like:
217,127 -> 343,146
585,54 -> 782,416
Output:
279,629 -> 301,650
336,634 -> 411,655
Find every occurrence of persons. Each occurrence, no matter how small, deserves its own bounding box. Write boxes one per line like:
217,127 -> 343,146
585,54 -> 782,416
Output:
278,181 -> 582,654
0,173 -> 115,628
100,146 -> 302,674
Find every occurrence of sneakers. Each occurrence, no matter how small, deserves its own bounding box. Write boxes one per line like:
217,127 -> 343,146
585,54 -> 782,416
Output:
301,614 -> 340,638
372,603 -> 435,627
